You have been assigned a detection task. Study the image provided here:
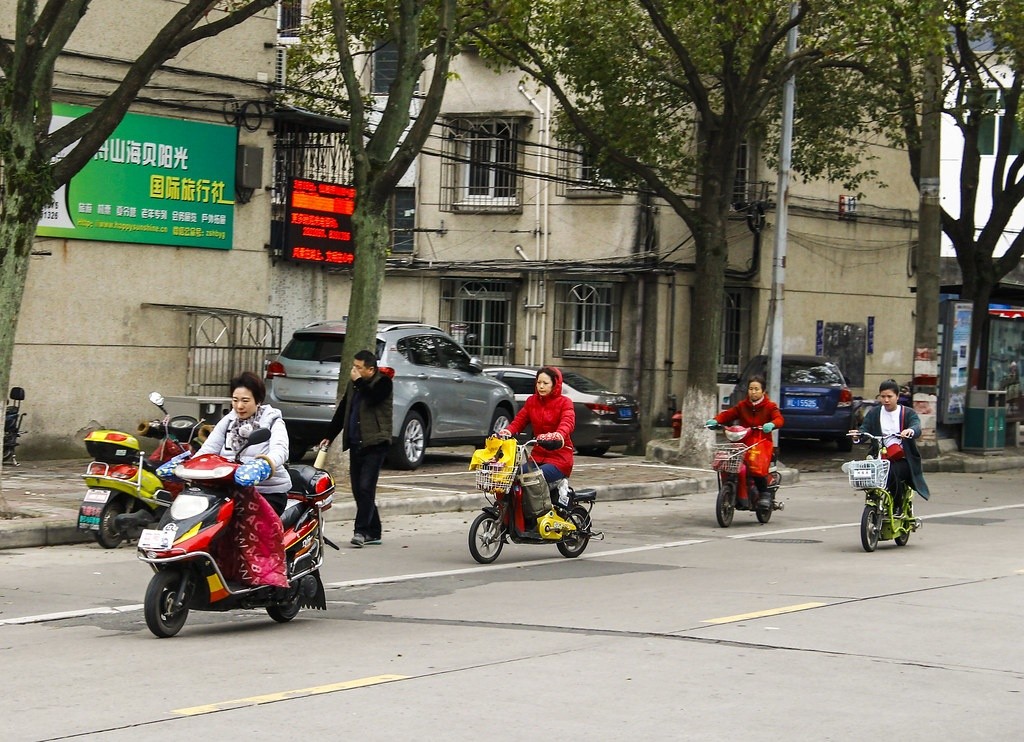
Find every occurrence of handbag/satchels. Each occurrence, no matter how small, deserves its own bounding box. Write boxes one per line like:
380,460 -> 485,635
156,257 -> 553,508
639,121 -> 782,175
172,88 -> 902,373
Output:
520,445 -> 552,515
882,443 -> 904,461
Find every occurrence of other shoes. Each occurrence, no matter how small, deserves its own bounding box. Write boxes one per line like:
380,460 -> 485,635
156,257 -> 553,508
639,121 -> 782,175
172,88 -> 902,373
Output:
351,533 -> 364,546
894,507 -> 902,516
758,497 -> 772,511
364,535 -> 382,544
525,518 -> 538,533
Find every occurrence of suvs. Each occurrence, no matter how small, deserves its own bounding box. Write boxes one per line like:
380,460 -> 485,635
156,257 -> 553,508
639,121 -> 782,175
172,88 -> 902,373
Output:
261,319 -> 519,470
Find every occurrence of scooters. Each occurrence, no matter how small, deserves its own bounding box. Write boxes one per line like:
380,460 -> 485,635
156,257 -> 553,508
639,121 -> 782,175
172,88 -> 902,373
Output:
704,423 -> 785,528
846,430 -> 922,551
137,428 -> 340,638
469,431 -> 604,564
3,387 -> 28,467
76,392 -> 216,549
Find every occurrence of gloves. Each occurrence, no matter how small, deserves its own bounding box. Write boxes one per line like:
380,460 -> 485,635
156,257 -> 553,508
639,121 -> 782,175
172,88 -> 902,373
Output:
706,419 -> 718,427
763,422 -> 775,433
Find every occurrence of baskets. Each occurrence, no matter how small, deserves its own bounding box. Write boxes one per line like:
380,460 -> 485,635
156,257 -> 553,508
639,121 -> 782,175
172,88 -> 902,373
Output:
476,463 -> 519,494
847,460 -> 891,489
711,443 -> 748,474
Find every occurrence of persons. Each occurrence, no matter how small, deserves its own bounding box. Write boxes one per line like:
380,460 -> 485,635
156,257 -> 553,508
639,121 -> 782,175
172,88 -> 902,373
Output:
495,366 -> 575,529
706,373 -> 784,508
192,371 -> 292,517
849,378 -> 930,516
320,350 -> 394,547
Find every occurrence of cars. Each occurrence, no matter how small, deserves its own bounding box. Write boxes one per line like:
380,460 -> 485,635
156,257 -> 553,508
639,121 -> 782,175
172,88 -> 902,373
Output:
730,355 -> 854,453
482,364 -> 642,457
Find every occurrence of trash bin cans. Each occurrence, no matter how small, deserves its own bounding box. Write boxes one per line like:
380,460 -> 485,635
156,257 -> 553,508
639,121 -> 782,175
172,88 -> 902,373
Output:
163,396 -> 234,427
961,390 -> 1007,456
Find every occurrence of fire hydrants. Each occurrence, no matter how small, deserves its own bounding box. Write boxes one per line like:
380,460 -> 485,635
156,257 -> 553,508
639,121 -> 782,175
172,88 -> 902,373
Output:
673,411 -> 682,438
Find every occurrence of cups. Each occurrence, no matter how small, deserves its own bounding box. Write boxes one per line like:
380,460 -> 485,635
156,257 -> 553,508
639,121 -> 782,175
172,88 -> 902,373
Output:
313,444 -> 329,470
